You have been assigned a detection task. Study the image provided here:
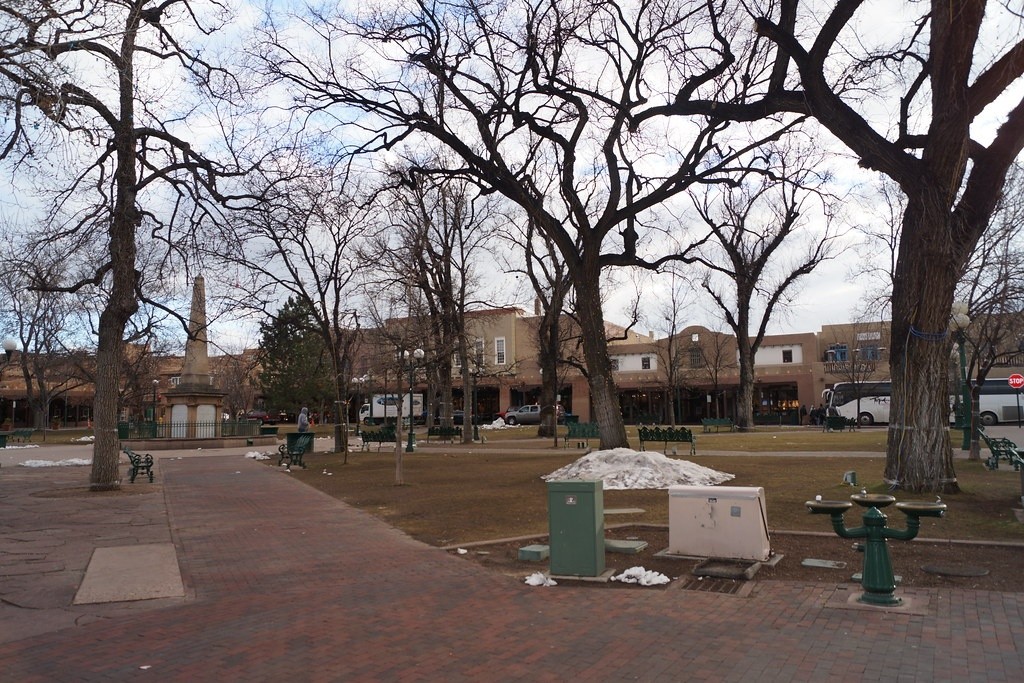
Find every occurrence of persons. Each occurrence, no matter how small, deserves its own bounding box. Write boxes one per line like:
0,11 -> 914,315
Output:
800,403 -> 826,426
128,410 -> 144,423
297,407 -> 309,433
238,411 -> 243,422
308,408 -> 335,425
402,414 -> 410,425
158,414 -> 164,427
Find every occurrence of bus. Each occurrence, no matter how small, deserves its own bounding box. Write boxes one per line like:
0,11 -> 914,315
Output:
822,378 -> 1024,428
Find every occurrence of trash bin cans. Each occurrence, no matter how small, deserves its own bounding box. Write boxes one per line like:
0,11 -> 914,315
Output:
955,403 -> 964,429
334,424 -> 345,453
117,420 -> 129,439
564,414 -> 579,425
826,416 -> 846,429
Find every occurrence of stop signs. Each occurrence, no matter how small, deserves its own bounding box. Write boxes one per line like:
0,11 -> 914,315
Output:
1008,374 -> 1024,388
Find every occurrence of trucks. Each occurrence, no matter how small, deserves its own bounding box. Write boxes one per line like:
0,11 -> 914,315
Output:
359,393 -> 423,426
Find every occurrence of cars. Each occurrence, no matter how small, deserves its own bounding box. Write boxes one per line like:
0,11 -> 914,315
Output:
495,410 -> 506,420
433,409 -> 475,424
242,409 -> 281,425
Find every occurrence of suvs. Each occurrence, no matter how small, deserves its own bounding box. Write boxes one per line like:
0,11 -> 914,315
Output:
504,405 -> 565,425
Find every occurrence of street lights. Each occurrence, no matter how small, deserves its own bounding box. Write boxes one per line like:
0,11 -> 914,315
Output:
351,377 -> 364,436
948,300 -> 971,449
151,380 -> 159,438
394,349 -> 425,452
666,317 -> 681,424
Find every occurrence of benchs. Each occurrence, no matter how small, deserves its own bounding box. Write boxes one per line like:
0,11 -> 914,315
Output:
427,426 -> 463,446
10,427 -> 37,443
822,416 -> 857,434
401,418 -> 417,430
123,444 -> 154,483
637,426 -> 696,456
360,429 -> 399,453
564,421 -> 604,448
278,433 -> 313,469
975,425 -> 1024,470
701,417 -> 737,433
